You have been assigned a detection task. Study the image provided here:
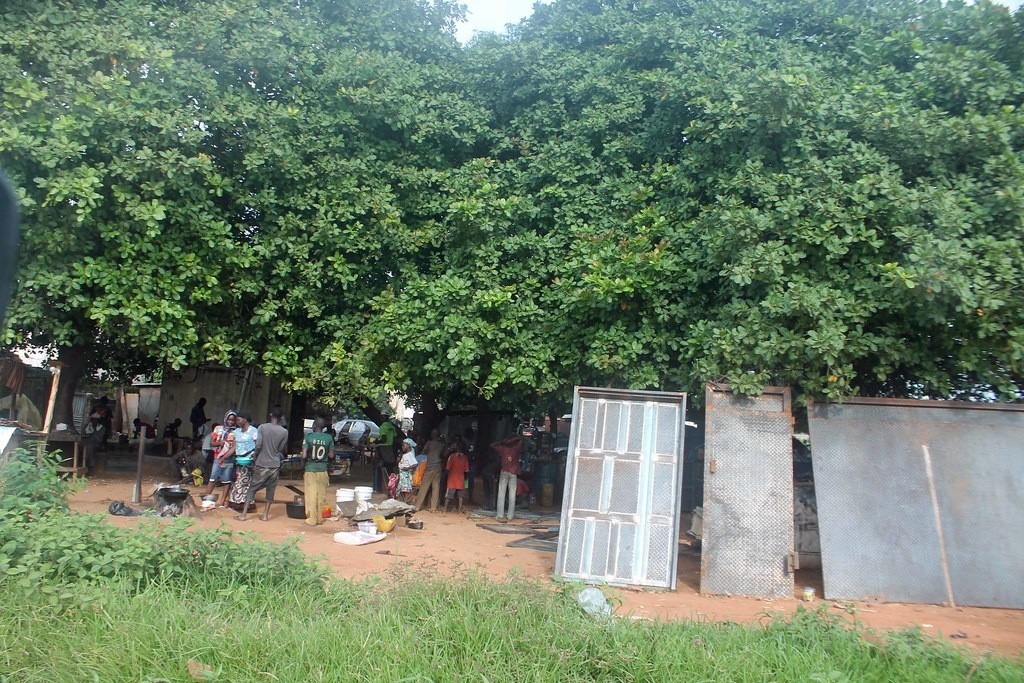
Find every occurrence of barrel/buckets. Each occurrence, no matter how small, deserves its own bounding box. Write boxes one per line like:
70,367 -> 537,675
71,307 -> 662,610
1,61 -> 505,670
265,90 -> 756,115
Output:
355,486 -> 373,501
357,522 -> 377,536
336,488 -> 355,511
534,461 -> 556,506
803,587 -> 816,602
322,506 -> 331,518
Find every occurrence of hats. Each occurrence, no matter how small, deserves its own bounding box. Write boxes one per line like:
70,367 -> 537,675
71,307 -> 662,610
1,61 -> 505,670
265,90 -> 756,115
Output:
381,409 -> 392,417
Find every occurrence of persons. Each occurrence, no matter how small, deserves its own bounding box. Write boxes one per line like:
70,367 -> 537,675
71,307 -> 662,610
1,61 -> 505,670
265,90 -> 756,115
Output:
370,409 -> 524,522
133,418 -> 156,439
84,396 -> 114,476
163,397 -> 335,525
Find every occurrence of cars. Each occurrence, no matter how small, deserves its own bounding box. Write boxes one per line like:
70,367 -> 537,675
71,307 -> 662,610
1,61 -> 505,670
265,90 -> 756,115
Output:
522,428 -> 533,437
322,419 -> 380,447
304,419 -> 315,435
554,445 -> 568,462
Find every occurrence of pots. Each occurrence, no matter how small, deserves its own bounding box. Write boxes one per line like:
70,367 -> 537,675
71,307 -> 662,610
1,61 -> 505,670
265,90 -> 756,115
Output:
159,488 -> 189,505
285,502 -> 307,519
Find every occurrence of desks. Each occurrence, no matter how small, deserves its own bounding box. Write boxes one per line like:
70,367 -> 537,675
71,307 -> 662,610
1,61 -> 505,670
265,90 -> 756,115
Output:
45,433 -> 90,483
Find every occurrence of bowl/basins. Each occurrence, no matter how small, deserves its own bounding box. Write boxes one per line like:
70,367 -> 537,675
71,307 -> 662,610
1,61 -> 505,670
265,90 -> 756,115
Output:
236,457 -> 253,465
191,493 -> 220,503
